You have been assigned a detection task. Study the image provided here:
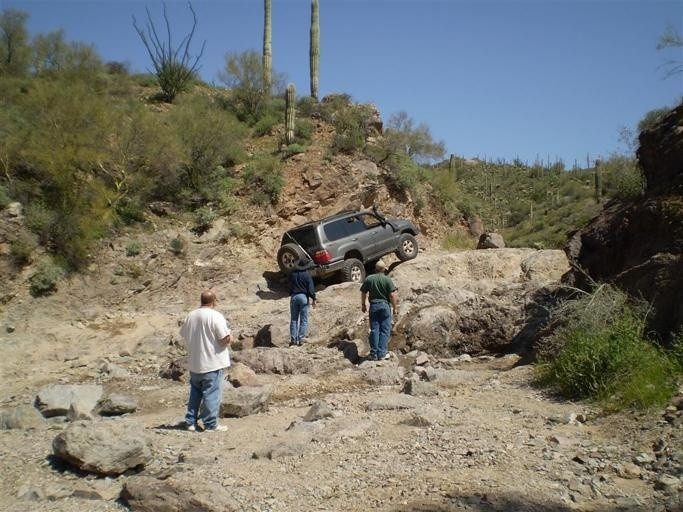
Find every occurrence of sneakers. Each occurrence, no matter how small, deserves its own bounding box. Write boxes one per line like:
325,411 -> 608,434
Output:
370,352 -> 391,360
289,342 -> 304,346
203,424 -> 229,433
184,423 -> 204,432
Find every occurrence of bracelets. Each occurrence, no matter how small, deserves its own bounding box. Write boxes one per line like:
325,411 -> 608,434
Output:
361,304 -> 364,306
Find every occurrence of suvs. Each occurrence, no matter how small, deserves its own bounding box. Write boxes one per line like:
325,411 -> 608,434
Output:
277,205 -> 419,283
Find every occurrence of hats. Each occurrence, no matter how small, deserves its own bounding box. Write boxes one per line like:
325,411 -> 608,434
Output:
293,258 -> 313,270
375,260 -> 386,272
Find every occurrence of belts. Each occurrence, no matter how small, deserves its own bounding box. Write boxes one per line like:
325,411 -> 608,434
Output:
370,300 -> 390,305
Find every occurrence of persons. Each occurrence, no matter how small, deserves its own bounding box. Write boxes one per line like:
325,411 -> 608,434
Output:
288,258 -> 316,345
359,261 -> 397,360
178,290 -> 233,431
